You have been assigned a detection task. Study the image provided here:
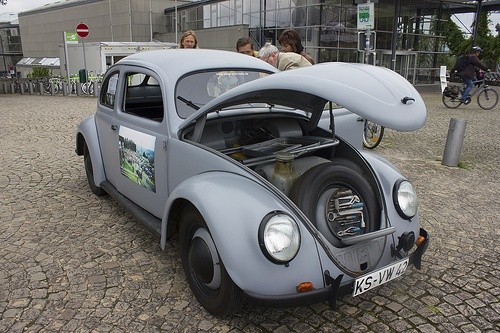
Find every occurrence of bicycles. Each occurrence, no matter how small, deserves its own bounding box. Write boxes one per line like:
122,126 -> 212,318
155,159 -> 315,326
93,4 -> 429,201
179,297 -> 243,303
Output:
363,118 -> 385,149
442,71 -> 499,110
16,71 -> 102,95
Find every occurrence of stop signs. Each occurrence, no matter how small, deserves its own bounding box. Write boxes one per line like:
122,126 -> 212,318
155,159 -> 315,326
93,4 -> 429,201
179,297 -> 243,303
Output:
76,23 -> 90,38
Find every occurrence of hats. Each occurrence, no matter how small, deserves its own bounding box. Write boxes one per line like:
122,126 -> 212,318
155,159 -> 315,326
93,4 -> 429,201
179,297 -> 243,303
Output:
472,46 -> 484,51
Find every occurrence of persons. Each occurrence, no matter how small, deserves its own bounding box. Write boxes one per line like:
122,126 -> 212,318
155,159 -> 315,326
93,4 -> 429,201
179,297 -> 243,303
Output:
279,30 -> 314,65
458,47 -> 491,102
236,38 -> 269,85
180,31 -> 197,49
257,43 -> 312,71
477,69 -> 486,88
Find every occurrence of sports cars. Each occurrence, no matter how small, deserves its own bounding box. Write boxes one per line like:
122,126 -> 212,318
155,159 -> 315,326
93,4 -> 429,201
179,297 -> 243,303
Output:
74,48 -> 431,318
